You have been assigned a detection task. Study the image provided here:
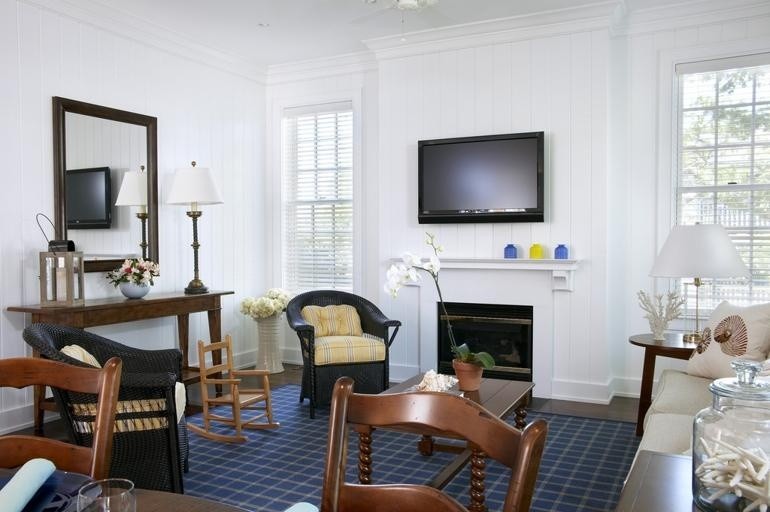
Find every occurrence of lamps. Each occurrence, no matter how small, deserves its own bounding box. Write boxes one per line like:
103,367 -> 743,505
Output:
648,222 -> 753,343
166,161 -> 225,294
115,166 -> 148,258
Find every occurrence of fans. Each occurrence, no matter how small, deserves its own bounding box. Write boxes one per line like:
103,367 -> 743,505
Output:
348,0 -> 444,38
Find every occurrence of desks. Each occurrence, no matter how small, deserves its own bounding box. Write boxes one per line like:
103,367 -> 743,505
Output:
614,451 -> 702,512
0,488 -> 251,512
629,330 -> 705,436
7,290 -> 235,439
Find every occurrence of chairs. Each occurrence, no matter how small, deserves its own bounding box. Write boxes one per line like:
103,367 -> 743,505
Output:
319,377 -> 548,512
0,356 -> 123,487
286,289 -> 401,419
23,322 -> 189,495
186,334 -> 279,443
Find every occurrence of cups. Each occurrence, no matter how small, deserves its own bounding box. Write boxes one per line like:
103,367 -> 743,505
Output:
78,478 -> 137,512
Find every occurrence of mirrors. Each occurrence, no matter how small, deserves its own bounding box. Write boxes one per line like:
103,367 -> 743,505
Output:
52,95 -> 159,274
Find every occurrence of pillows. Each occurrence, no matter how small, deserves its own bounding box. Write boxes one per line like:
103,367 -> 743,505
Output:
301,303 -> 365,337
61,344 -> 103,369
686,300 -> 770,381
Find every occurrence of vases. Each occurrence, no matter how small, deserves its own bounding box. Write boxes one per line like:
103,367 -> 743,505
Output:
120,281 -> 150,299
453,358 -> 485,392
255,315 -> 286,374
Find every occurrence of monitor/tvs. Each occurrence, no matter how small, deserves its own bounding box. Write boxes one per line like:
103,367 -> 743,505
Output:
418,130 -> 545,225
64,164 -> 114,230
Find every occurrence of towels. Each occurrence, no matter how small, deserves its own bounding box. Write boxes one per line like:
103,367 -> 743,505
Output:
284,501 -> 320,512
0,457 -> 55,512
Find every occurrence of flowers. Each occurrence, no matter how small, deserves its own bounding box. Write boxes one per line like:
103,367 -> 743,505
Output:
383,231 -> 499,362
107,258 -> 162,282
239,288 -> 296,321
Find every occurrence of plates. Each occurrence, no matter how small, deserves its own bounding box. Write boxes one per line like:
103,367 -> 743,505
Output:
0,470 -> 104,512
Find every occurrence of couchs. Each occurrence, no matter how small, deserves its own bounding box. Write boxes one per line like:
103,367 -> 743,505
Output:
619,369 -> 770,489
